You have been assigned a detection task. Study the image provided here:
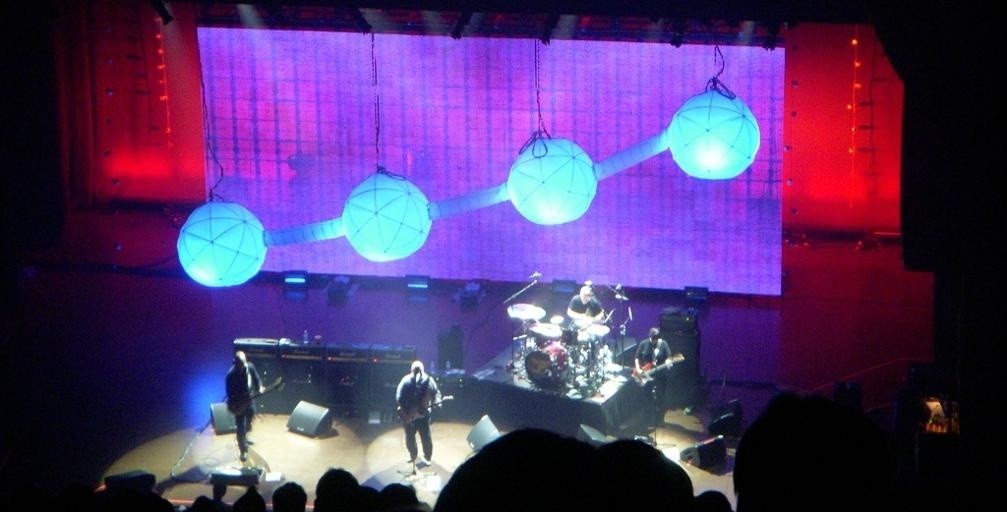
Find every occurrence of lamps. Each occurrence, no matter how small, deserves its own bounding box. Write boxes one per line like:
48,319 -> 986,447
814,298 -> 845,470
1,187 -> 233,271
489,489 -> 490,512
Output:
177,0 -> 761,289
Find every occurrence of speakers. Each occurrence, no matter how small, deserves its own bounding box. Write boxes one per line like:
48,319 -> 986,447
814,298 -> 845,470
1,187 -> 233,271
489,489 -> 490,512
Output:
467,414 -> 500,454
288,400 -> 332,437
680,434 -> 726,470
576,422 -> 608,450
103,471 -> 157,492
708,398 -> 742,434
209,401 -> 238,434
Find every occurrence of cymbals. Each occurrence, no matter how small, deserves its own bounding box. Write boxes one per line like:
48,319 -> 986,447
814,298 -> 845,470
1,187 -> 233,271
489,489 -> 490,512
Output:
507,303 -> 546,320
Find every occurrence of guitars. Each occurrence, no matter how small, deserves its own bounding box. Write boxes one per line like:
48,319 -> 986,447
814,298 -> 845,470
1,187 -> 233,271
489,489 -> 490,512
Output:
633,354 -> 684,388
227,379 -> 284,416
396,394 -> 455,423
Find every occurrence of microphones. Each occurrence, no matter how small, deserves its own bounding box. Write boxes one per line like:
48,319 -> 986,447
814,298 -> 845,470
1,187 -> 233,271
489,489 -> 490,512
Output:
415,372 -> 420,385
606,283 -> 618,294
529,272 -> 541,280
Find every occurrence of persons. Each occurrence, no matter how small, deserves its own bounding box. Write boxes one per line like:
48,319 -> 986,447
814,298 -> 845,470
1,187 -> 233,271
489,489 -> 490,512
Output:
632,327 -> 672,428
224,350 -> 265,460
567,285 -> 605,325
0,386 -> 1006,512
396,359 -> 442,466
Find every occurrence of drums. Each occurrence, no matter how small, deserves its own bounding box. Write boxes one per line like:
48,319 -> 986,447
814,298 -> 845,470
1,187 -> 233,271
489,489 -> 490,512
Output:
562,328 -> 577,344
577,326 -> 610,351
525,343 -> 571,385
526,326 -> 564,344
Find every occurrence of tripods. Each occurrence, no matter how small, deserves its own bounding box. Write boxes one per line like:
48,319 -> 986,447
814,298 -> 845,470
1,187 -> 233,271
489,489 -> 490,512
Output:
607,294 -> 635,380
396,385 -> 435,483
494,280 -> 537,382
646,363 -> 676,449
